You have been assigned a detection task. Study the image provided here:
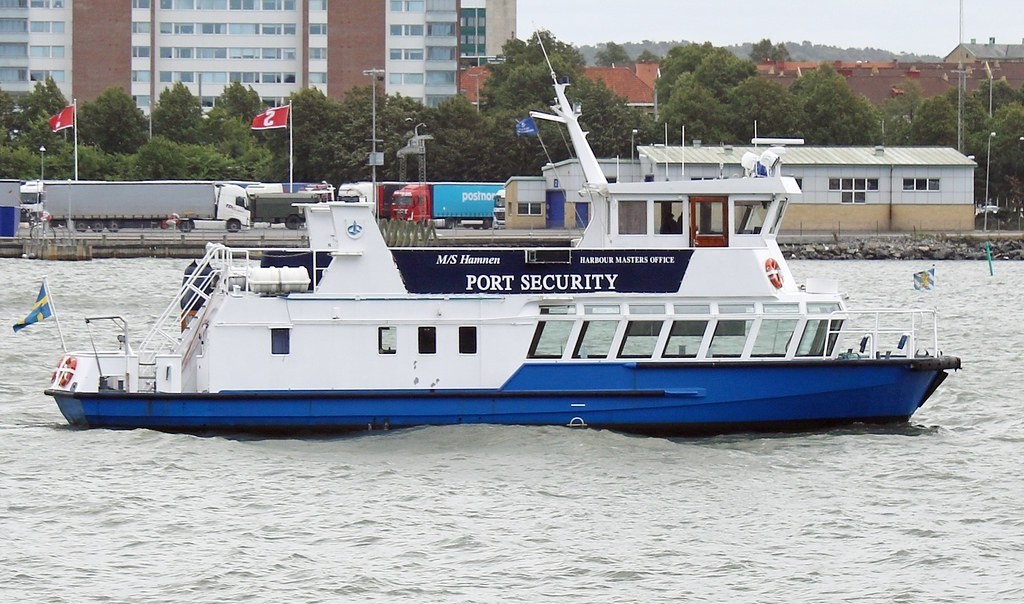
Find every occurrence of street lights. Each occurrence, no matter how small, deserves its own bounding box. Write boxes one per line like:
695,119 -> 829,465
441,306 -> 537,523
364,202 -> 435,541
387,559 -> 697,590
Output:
631,129 -> 638,182
39,146 -> 46,179
983,132 -> 997,231
362,69 -> 386,223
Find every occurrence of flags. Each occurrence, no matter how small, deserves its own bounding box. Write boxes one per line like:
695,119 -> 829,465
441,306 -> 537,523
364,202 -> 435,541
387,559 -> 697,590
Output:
515,117 -> 538,138
250,104 -> 290,130
912,267 -> 934,291
12,282 -> 54,334
49,104 -> 74,133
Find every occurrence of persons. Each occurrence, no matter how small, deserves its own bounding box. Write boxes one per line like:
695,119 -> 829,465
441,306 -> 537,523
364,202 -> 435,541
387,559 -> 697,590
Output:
658,211 -> 684,235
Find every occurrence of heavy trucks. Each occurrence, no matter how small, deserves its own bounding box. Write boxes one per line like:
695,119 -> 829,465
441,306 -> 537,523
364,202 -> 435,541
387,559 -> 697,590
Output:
41,179 -> 251,234
390,181 -> 505,230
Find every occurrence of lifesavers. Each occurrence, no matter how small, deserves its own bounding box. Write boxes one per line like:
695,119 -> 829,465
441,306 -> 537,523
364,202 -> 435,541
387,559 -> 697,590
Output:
49,355 -> 77,387
764,258 -> 785,288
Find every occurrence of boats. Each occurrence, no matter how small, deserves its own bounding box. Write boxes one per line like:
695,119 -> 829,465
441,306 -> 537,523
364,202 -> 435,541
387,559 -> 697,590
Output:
44,19 -> 962,439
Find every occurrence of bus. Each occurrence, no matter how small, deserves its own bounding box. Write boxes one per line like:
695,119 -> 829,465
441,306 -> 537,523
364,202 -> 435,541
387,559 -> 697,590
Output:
492,189 -> 507,230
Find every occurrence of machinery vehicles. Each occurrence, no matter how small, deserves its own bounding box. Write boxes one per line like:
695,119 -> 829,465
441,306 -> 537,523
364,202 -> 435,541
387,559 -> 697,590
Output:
235,177 -> 377,230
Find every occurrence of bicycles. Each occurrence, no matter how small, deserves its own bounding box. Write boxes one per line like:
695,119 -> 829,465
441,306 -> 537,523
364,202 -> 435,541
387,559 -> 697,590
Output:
19,205 -> 57,247
